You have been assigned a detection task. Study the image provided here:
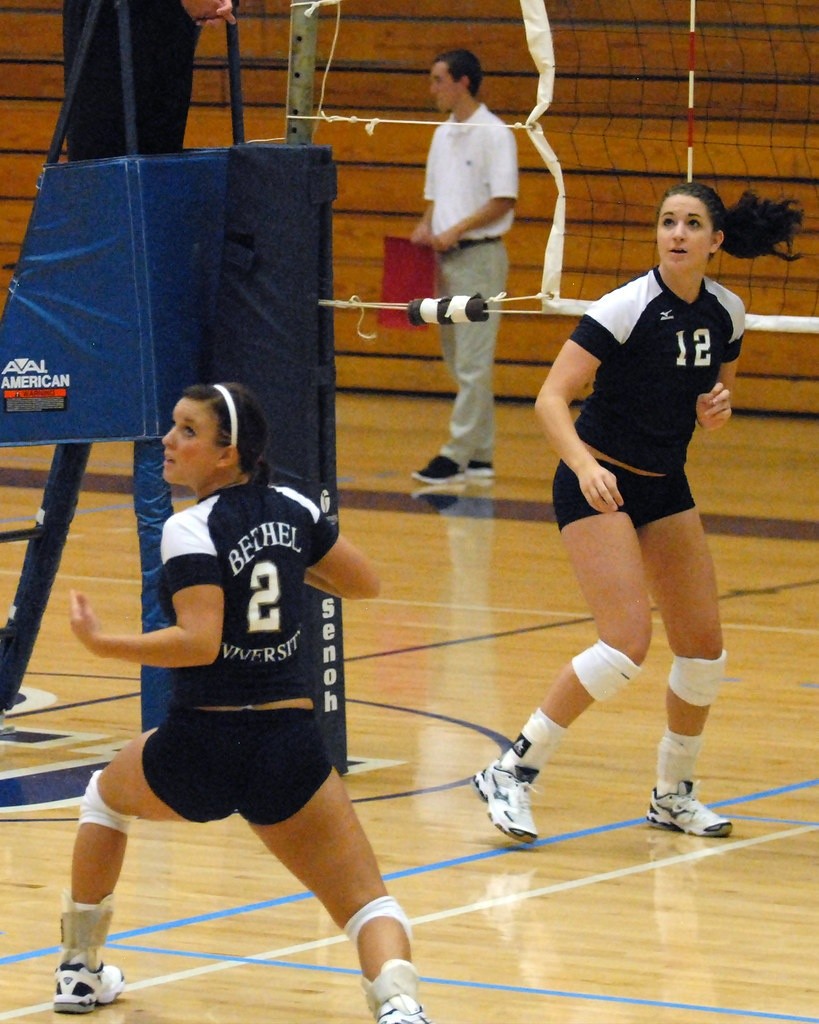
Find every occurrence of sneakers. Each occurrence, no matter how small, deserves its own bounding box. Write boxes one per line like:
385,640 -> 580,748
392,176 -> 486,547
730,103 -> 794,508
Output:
645,779 -> 733,837
375,993 -> 429,1024
471,757 -> 540,845
411,454 -> 497,486
53,960 -> 125,1014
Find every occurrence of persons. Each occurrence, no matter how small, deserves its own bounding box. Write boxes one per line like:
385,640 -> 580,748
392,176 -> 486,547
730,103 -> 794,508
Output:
411,48 -> 518,484
61,0 -> 236,161
469,182 -> 802,844
53,382 -> 433,1024
